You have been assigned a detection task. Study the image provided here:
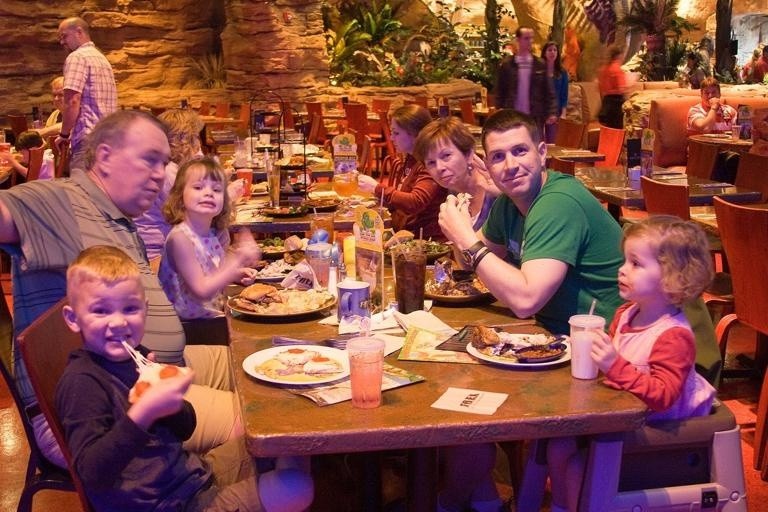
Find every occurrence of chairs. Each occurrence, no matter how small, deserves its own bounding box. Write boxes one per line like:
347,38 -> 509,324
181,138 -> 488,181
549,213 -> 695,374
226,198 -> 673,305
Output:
18,295 -> 92,510
735,154 -> 760,196
235,103 -> 253,141
458,97 -> 478,124
372,99 -> 395,171
303,101 -> 332,147
405,97 -> 432,121
342,102 -> 386,170
49,132 -> 70,179
5,114 -> 31,142
639,175 -> 694,220
685,143 -> 721,176
218,102 -> 232,122
277,94 -> 297,131
595,126 -> 627,169
24,144 -> 45,185
253,99 -> 283,148
710,196 -> 762,400
200,99 -> 214,123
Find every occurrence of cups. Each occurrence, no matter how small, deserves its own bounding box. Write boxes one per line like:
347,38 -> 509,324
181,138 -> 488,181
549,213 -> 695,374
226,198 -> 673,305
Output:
310,213 -> 333,245
237,168 -> 253,196
234,141 -> 246,167
731,126 -> 742,141
391,250 -> 424,313
305,242 -> 332,287
628,168 -> 641,190
336,281 -> 371,323
245,137 -> 257,155
346,337 -> 385,409
1,143 -> 10,166
568,315 -> 606,380
266,160 -> 279,206
332,162 -> 360,218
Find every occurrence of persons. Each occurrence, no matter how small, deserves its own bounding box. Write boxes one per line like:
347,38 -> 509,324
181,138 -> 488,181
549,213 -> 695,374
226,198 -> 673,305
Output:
128,108 -> 201,262
0,108 -> 241,470
681,50 -> 705,89
742,48 -> 762,81
53,244 -> 315,512
2,130 -> 56,180
437,109 -> 629,511
494,26 -> 557,144
54,18 -> 117,175
686,77 -> 737,163
541,40 -> 569,144
157,157 -> 262,321
547,213 -> 715,511
415,120 -> 501,235
40,77 -> 64,136
358,104 -> 448,242
597,47 -> 638,130
752,45 -> 768,83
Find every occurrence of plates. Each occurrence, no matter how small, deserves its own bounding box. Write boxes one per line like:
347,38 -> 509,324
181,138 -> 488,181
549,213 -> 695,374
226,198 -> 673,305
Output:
271,160 -> 304,169
256,127 -> 274,132
385,241 -> 454,260
228,238 -> 303,256
424,288 -> 493,306
226,288 -> 336,317
302,200 -> 342,211
242,344 -> 350,385
466,333 -> 572,367
263,206 -> 308,217
253,274 -> 285,281
280,188 -> 307,197
254,146 -> 277,151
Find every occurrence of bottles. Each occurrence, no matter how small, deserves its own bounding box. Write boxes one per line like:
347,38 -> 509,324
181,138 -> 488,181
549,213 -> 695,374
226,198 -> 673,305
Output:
340,263 -> 347,282
328,260 -> 338,297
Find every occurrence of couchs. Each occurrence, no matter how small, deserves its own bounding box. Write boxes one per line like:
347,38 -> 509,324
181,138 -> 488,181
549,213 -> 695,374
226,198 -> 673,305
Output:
647,98 -> 763,172
568,80 -> 680,148
624,86 -> 764,156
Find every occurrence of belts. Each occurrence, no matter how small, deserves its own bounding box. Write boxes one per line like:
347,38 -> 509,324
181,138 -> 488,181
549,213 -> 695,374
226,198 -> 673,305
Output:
24,402 -> 42,421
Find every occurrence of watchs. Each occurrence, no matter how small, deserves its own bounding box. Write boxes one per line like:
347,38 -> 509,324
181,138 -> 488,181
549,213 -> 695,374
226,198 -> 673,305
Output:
458,239 -> 485,266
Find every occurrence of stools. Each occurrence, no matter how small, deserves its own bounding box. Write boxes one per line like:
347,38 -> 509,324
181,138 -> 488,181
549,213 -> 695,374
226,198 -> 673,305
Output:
513,397 -> 748,511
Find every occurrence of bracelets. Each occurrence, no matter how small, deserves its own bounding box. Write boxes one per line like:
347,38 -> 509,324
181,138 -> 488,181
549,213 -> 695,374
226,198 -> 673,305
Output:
471,247 -> 494,270
